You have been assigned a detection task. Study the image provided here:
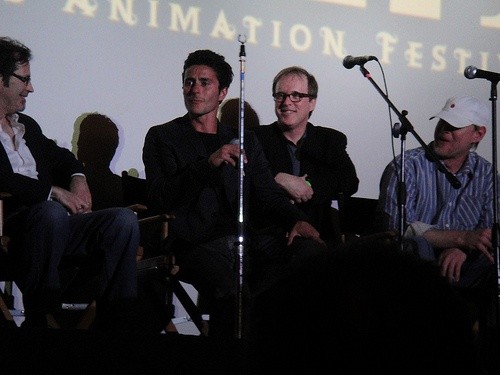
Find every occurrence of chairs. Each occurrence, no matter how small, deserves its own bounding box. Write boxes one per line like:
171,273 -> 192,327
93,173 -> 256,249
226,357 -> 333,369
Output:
123,171 -> 213,335
0,191 -> 149,329
336,194 -> 397,265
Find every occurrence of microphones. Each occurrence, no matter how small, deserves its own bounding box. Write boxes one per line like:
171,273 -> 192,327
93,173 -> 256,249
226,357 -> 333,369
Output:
464,66 -> 500,82
343,55 -> 374,69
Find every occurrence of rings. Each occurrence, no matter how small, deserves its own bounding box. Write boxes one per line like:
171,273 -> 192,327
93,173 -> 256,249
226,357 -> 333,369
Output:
76,206 -> 83,210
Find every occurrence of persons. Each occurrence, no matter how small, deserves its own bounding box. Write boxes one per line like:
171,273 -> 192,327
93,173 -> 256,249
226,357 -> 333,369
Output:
377,96 -> 500,343
247,66 -> 400,335
141,49 -> 328,347
0,36 -> 142,331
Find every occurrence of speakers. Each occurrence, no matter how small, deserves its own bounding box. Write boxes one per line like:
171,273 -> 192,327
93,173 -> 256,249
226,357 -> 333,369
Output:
251,246 -> 429,345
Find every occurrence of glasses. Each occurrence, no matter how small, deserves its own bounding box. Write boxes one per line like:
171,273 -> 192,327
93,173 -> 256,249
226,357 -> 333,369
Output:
9,73 -> 31,83
272,91 -> 313,102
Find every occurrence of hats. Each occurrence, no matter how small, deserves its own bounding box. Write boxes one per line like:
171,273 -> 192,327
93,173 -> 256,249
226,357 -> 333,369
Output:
428,95 -> 489,128
